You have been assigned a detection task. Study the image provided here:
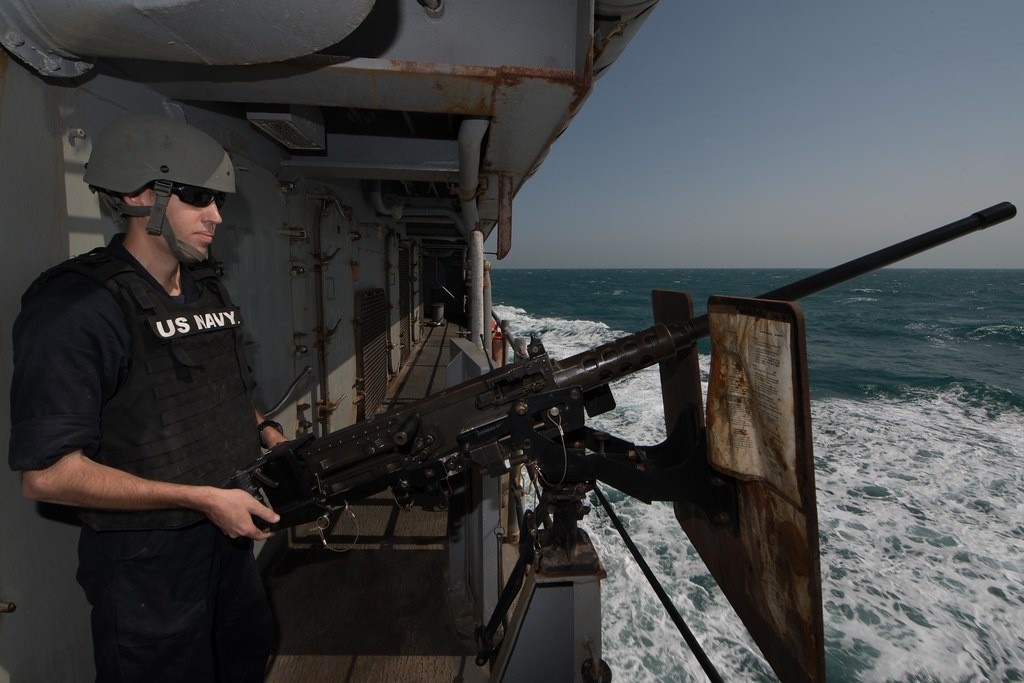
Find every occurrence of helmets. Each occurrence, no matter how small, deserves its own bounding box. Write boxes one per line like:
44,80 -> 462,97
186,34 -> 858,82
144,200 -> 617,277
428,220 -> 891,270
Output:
83,114 -> 235,193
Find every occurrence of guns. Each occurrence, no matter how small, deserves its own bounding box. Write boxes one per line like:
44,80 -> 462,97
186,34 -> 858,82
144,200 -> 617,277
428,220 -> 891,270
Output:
211,197 -> 1023,551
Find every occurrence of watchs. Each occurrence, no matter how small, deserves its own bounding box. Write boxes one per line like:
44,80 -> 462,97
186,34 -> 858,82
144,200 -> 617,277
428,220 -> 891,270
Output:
259,420 -> 284,449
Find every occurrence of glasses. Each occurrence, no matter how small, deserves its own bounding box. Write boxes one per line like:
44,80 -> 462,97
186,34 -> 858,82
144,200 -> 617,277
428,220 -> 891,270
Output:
150,186 -> 228,209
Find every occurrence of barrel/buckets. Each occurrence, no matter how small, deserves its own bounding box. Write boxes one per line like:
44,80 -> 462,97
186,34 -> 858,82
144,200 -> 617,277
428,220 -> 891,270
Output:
432,305 -> 444,322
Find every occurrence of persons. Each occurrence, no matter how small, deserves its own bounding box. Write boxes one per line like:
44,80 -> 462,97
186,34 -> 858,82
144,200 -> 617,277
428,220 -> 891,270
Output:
10,115 -> 289,683
